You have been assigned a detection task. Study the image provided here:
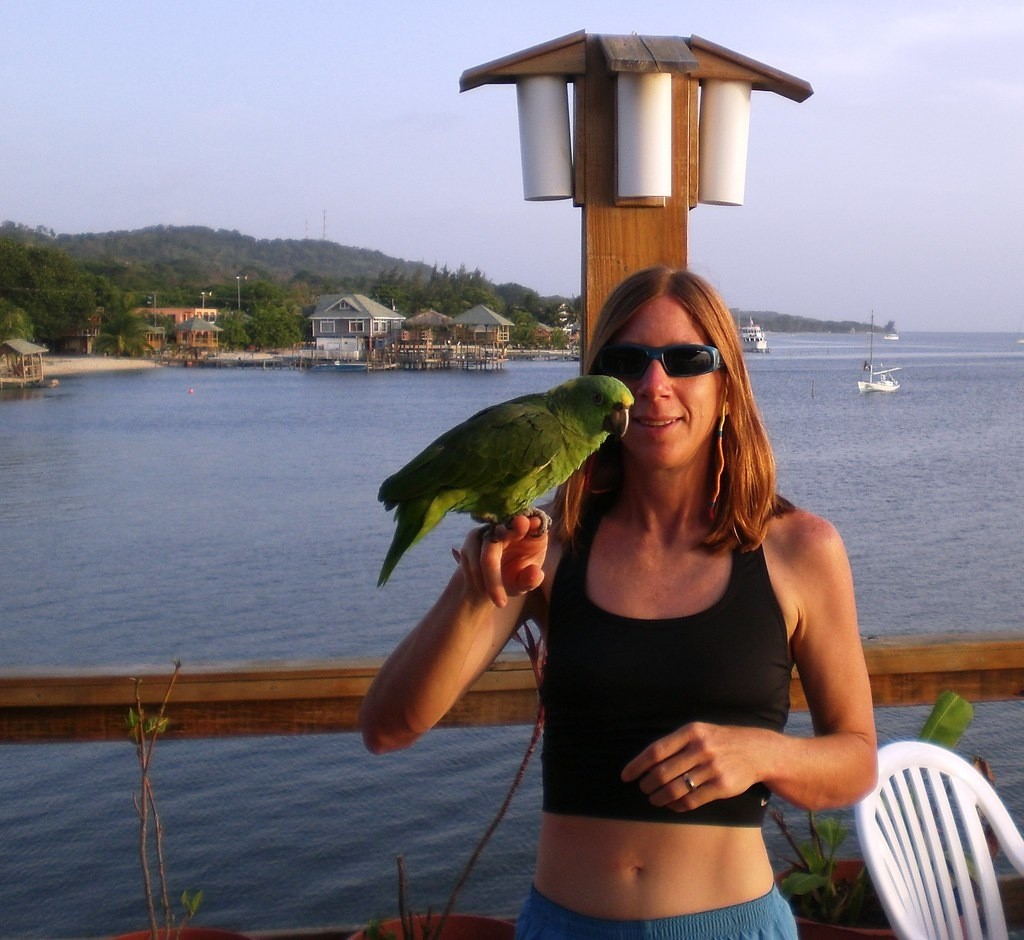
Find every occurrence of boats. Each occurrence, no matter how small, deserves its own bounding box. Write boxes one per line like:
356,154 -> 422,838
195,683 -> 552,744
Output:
738,316 -> 770,354
311,364 -> 372,372
884,334 -> 900,340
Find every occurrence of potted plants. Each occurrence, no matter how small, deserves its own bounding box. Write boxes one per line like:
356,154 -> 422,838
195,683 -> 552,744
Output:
346,547 -> 549,940
774,690 -> 1000,940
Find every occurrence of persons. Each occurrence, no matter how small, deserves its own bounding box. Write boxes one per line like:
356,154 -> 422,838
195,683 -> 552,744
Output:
969,755 -> 1000,864
360,268 -> 878,940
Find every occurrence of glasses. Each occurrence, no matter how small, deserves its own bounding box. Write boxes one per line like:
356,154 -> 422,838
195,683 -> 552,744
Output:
598,343 -> 721,379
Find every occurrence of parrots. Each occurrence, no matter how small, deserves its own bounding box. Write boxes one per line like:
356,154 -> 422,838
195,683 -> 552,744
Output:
372,375 -> 636,590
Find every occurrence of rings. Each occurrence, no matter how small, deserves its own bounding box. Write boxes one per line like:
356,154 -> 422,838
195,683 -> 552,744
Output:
683,774 -> 696,791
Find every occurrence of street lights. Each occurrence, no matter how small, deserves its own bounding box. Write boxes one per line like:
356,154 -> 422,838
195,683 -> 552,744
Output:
201,292 -> 205,315
152,292 -> 156,326
237,277 -> 240,309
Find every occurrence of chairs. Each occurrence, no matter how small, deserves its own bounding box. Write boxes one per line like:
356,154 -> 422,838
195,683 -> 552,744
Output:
854,740 -> 1024,940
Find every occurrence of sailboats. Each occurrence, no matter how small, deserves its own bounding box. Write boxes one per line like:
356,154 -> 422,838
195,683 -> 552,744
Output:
857,309 -> 903,393
